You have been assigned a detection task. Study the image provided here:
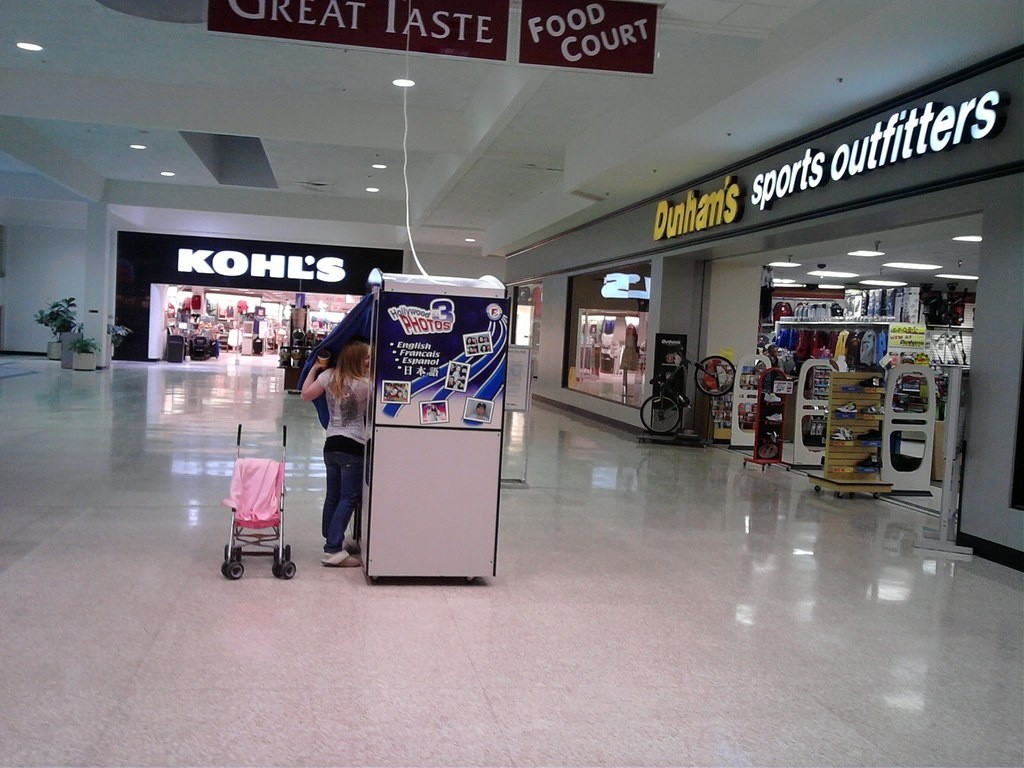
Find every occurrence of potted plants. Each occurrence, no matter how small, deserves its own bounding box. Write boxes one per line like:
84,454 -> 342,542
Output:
34,297 -> 127,371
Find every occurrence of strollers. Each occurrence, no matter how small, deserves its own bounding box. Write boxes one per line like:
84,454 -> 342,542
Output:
221,424 -> 297,581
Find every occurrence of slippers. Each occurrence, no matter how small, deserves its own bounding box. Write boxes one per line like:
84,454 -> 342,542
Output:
321,549 -> 360,567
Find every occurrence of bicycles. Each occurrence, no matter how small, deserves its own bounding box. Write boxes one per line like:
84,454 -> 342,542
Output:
639,342 -> 737,434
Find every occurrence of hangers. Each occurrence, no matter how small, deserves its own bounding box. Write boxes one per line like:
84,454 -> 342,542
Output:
778,321 -> 886,333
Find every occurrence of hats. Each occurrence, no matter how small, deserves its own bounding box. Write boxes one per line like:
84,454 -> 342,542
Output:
477,403 -> 487,409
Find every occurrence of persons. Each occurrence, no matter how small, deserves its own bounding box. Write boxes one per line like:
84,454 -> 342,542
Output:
757,344 -> 785,375
448,362 -> 468,390
384,383 -> 409,402
468,403 -> 489,421
300,340 -> 385,568
466,335 -> 491,355
426,403 -> 441,422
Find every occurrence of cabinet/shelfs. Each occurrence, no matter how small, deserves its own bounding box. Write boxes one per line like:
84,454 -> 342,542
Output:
830,388 -> 885,476
763,388 -> 789,447
